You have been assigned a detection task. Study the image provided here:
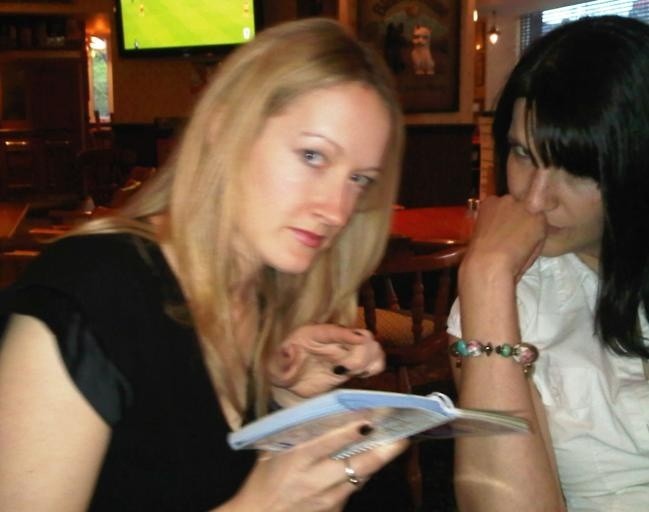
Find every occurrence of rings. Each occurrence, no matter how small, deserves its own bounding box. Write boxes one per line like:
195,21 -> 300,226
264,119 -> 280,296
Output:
344,460 -> 362,488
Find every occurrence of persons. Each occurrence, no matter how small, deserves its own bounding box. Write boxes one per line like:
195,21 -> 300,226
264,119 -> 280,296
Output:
444,13 -> 649,512
0,16 -> 410,511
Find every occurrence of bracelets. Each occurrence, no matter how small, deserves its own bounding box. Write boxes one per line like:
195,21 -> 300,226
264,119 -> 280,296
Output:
449,338 -> 539,366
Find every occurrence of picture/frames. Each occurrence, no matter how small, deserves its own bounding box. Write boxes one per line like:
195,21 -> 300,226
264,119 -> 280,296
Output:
337,0 -> 479,129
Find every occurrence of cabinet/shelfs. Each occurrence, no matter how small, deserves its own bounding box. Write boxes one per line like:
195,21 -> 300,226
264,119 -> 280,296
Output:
0,2 -> 85,64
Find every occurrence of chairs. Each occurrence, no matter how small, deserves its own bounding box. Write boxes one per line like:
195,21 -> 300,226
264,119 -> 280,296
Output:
352,246 -> 471,504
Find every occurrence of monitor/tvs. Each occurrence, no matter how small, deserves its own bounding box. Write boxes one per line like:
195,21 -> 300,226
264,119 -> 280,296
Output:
115,0 -> 260,59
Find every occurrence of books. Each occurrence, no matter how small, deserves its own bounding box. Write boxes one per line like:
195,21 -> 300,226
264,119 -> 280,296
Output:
226,387 -> 530,453
